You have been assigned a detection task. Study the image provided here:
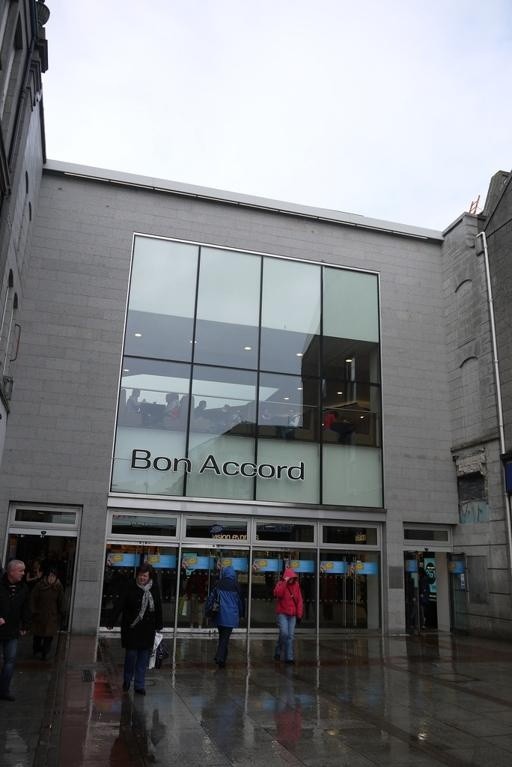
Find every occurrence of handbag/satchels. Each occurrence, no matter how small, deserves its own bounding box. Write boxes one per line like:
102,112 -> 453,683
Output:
296,604 -> 305,624
158,641 -> 169,659
205,596 -> 220,617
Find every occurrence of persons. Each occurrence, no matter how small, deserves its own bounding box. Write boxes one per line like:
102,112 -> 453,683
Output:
204,565 -> 245,670
274,670 -> 303,753
107,562 -> 163,695
407,571 -> 416,628
0,559 -> 30,702
206,675 -> 247,750
303,573 -> 357,619
25,561 -> 44,588
272,567 -> 305,665
419,568 -> 431,630
185,568 -> 209,629
125,385 -> 300,440
25,566 -> 67,663
110,699 -> 157,767
323,411 -> 342,442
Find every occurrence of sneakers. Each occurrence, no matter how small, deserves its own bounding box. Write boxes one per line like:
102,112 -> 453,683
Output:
2,692 -> 17,702
33,652 -> 48,661
276,654 -> 296,665
215,659 -> 226,667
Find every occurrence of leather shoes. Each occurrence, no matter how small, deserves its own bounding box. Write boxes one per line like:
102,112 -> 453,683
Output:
123,681 -> 146,694
